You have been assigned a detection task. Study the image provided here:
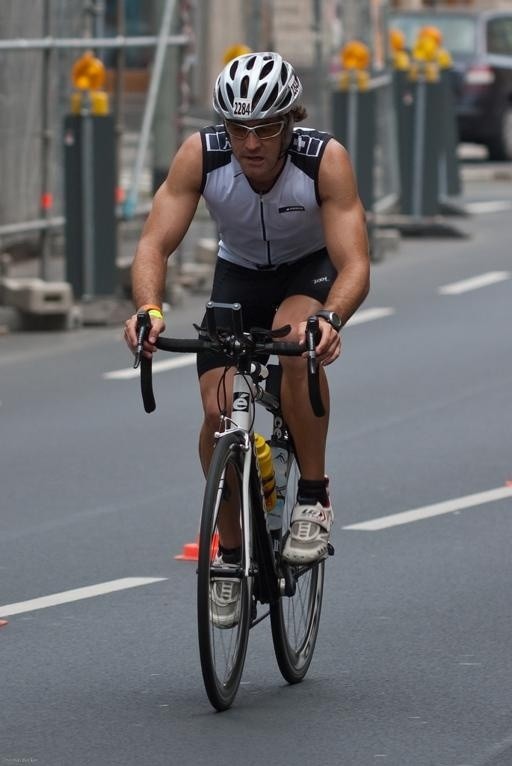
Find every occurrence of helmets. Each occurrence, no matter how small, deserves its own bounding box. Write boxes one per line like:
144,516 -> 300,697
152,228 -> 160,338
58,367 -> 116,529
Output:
212,52 -> 303,121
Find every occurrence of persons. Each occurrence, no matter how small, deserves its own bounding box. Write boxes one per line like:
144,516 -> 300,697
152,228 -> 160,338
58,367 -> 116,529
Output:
123,51 -> 371,630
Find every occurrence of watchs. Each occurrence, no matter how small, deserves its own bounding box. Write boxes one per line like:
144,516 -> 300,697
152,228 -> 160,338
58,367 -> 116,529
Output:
313,309 -> 343,332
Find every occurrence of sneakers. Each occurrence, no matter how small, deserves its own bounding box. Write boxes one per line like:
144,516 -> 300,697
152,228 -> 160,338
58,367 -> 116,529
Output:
282,488 -> 335,565
209,548 -> 243,629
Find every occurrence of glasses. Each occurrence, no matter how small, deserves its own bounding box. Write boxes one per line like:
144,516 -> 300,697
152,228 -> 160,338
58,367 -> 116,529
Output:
223,117 -> 287,140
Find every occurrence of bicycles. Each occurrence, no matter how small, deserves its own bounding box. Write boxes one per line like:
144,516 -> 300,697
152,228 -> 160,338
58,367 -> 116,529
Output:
134,312 -> 336,712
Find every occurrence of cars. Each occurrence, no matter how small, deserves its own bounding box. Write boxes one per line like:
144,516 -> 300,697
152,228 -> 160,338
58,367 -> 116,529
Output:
378,4 -> 510,162
